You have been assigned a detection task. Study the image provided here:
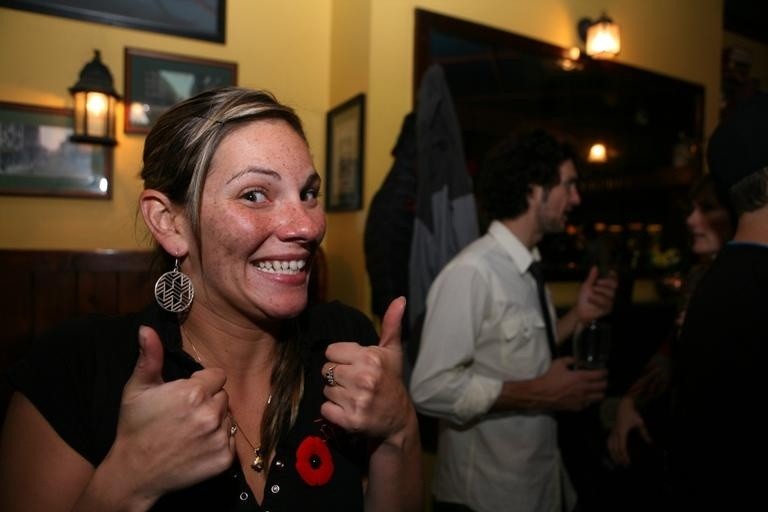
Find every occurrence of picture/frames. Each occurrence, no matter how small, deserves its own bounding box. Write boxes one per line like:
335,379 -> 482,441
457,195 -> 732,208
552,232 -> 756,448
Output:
124,46 -> 237,134
324,94 -> 364,212
0,100 -> 112,200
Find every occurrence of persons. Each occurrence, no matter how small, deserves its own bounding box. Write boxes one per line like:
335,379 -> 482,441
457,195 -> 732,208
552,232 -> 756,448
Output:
668,99 -> 768,512
1,87 -> 427,512
405,143 -> 621,511
607,174 -> 737,512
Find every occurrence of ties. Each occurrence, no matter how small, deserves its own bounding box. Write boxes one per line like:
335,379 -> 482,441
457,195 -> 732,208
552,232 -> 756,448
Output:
528,262 -> 561,363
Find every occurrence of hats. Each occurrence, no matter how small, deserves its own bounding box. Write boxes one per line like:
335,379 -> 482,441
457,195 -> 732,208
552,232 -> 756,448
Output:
703,93 -> 766,189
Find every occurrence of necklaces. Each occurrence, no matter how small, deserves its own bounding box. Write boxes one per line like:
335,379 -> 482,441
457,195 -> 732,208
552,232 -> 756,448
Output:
183,325 -> 271,473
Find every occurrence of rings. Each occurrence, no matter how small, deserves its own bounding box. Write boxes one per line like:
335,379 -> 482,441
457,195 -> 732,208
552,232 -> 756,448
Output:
228,413 -> 237,436
325,361 -> 338,386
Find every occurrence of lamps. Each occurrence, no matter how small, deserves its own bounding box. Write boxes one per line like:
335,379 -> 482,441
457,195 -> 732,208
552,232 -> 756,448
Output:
1,0 -> 226,43
577,12 -> 621,58
68,49 -> 119,147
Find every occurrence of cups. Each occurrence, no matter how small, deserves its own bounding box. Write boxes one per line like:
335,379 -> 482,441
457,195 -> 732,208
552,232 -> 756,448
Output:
572,317 -> 611,372
